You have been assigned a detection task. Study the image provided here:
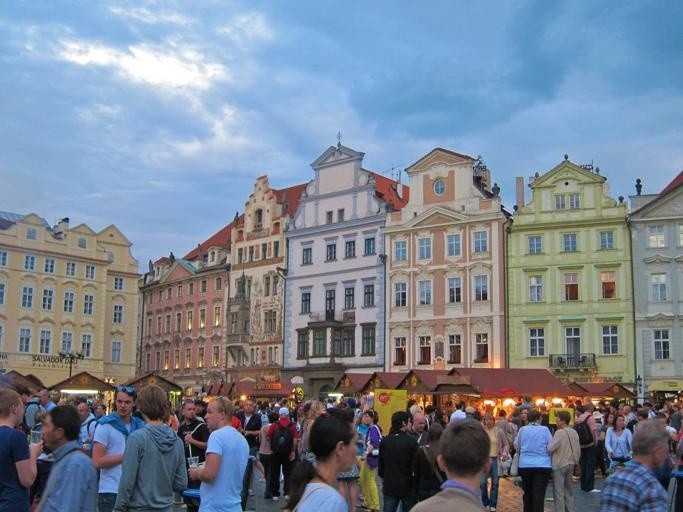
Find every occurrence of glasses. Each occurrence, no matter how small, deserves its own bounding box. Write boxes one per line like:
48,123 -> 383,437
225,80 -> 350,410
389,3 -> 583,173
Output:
216,396 -> 227,415
116,385 -> 135,394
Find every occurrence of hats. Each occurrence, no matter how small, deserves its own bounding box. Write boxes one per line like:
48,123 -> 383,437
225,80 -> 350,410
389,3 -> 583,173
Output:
592,411 -> 605,419
278,407 -> 290,417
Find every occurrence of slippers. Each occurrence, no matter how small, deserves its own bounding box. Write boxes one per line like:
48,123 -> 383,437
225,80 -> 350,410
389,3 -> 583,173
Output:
356,503 -> 366,508
366,507 -> 379,512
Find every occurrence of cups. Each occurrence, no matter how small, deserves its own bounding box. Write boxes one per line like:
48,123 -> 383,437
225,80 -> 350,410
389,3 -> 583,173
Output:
30,429 -> 42,444
187,456 -> 199,468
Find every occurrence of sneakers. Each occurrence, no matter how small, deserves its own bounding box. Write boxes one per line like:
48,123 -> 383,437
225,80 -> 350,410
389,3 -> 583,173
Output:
272,496 -> 279,501
248,488 -> 255,496
285,495 -> 291,500
484,504 -> 496,512
586,487 -> 602,493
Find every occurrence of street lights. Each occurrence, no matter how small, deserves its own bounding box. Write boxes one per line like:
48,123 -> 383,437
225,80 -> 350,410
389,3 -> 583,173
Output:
101,376 -> 115,384
58,350 -> 84,379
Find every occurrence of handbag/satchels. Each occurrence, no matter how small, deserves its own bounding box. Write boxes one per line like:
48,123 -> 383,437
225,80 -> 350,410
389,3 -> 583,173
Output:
509,449 -> 520,475
367,425 -> 382,456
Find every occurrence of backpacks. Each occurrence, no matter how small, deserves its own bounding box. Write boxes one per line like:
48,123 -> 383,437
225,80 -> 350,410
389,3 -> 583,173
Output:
573,414 -> 595,446
270,419 -> 295,457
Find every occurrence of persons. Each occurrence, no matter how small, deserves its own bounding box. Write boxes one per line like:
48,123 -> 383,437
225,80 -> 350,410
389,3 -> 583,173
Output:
412,417 -> 491,509
171,388 -> 382,509
4,388 -> 44,508
281,413 -> 358,509
114,384 -> 188,509
1,383 -> 106,458
188,396 -> 249,509
36,405 -> 97,508
600,419 -> 673,509
380,388 -> 682,509
92,385 -> 146,508
